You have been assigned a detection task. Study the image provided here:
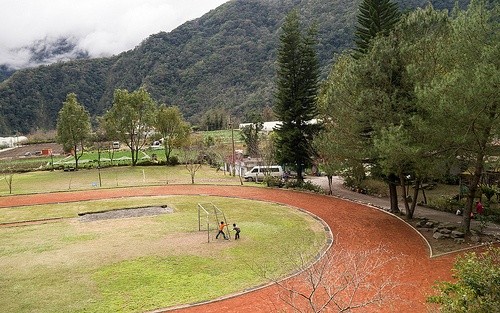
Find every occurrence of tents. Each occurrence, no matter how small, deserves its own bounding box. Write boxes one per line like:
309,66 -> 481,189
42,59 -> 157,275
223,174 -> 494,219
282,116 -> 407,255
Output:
456,155 -> 500,201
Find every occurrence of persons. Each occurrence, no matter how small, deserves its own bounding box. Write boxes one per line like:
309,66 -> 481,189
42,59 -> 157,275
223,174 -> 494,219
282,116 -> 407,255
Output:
475,200 -> 483,215
216,222 -> 229,239
282,167 -> 291,183
315,168 -> 320,177
230,223 -> 241,240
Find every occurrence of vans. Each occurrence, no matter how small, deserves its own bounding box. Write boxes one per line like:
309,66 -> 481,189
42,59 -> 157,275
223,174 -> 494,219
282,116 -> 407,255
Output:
244,165 -> 285,182
112,142 -> 120,149
